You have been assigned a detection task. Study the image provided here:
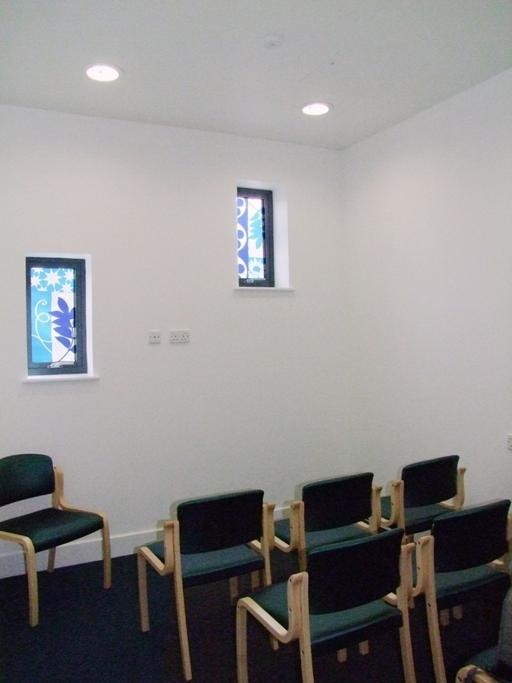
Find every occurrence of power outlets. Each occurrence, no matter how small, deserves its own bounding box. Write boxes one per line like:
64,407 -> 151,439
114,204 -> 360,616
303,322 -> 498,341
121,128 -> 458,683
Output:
149,330 -> 161,344
169,329 -> 181,342
180,330 -> 190,342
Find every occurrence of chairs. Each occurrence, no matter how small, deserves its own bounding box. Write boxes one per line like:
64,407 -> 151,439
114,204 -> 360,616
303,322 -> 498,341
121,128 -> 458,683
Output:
135,489 -> 279,681
363,455 -> 467,530
393,498 -> 512,683
251,471 -> 383,589
0,453 -> 112,628
456,647 -> 512,683
236,528 -> 417,683
